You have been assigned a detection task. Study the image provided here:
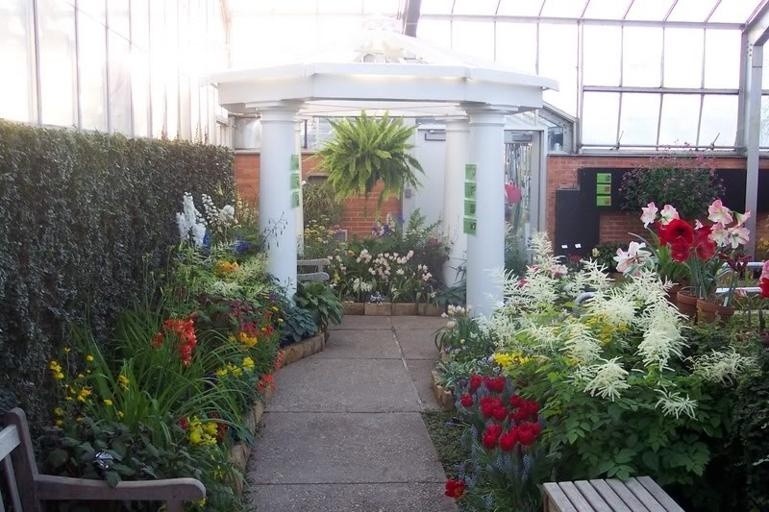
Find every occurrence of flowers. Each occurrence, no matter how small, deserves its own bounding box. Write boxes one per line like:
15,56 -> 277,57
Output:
637,200 -> 754,298
306,214 -> 446,314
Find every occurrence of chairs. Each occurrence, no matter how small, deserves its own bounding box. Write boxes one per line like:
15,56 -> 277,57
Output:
1,408 -> 205,512
